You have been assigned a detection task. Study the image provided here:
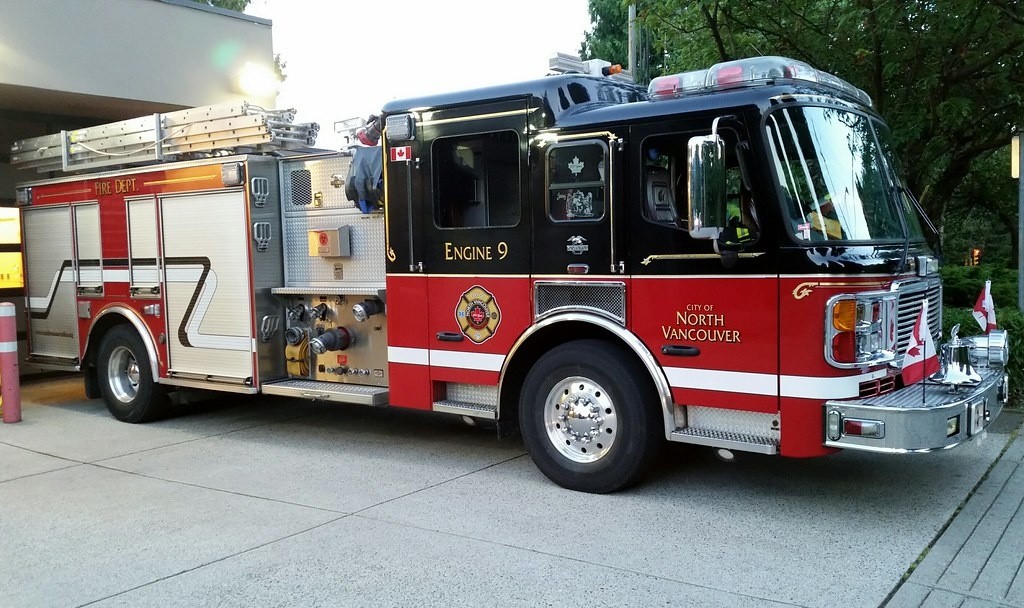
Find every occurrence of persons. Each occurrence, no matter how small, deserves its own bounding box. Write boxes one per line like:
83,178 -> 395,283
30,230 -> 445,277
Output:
721,183 -> 756,245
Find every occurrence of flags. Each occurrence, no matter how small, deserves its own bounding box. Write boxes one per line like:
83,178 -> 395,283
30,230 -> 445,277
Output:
901,297 -> 939,385
972,286 -> 997,332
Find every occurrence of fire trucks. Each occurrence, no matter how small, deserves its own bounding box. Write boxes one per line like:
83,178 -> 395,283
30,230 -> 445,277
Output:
10,6 -> 1013,492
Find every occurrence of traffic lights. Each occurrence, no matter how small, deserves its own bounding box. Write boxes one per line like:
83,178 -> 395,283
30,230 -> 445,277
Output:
972,248 -> 979,267
